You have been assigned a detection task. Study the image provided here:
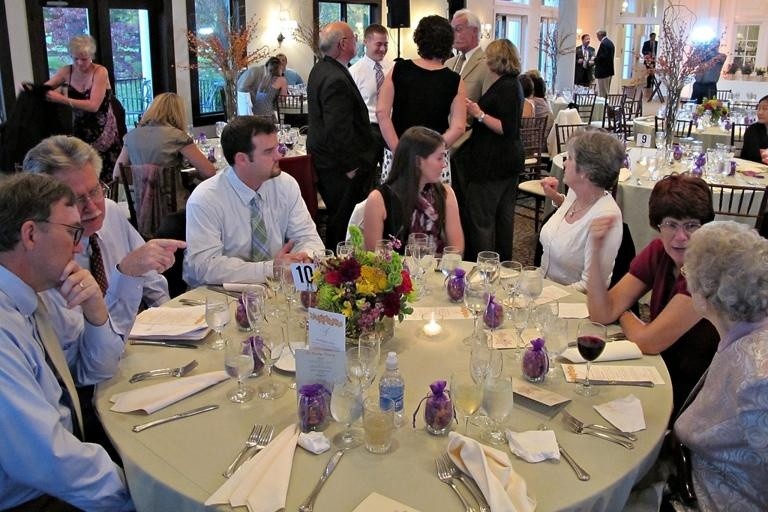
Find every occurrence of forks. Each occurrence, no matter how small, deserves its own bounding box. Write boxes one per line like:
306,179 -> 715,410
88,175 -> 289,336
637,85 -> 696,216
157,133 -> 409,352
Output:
440,450 -> 489,511
537,422 -> 590,483
434,457 -> 475,511
555,405 -> 640,452
222,423 -> 263,479
229,424 -> 274,479
128,359 -> 199,384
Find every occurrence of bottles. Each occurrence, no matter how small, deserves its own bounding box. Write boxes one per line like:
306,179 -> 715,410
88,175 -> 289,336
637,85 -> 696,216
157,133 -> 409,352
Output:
378,352 -> 404,426
520,338 -> 551,386
296,384 -> 330,433
424,381 -> 455,435
235,296 -> 254,333
444,268 -> 465,304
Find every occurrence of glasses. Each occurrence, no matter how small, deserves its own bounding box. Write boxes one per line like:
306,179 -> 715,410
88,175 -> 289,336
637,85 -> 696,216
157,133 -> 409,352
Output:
657,222 -> 701,235
338,34 -> 358,48
22,217 -> 84,247
72,180 -> 110,206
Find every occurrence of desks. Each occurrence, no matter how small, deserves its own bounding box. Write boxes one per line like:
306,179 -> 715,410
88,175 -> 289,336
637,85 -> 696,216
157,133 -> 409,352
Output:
93,251 -> 674,512
644,68 -> 668,106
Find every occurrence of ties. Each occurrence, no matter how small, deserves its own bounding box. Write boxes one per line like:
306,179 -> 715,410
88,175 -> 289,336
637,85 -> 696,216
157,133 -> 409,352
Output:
89,232 -> 109,299
374,63 -> 385,97
32,293 -> 85,442
454,54 -> 466,73
583,47 -> 589,69
249,193 -> 271,262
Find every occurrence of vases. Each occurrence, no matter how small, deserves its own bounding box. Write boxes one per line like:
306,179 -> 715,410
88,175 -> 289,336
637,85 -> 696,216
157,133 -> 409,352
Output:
654,79 -> 692,169
218,68 -> 242,124
549,56 -> 557,102
343,309 -> 396,347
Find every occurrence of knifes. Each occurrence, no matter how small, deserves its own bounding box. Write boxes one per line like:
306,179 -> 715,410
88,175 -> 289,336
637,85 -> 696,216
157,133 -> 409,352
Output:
299,448 -> 344,511
129,340 -> 199,349
131,404 -> 220,434
575,377 -> 654,388
568,328 -> 626,346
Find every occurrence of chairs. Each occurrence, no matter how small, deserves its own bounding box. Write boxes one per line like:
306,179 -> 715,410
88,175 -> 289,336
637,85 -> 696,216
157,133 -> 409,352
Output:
90,85 -> 320,246
517,80 -> 768,316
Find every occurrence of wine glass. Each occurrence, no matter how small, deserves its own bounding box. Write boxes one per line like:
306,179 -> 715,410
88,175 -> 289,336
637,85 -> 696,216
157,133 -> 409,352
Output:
336,241 -> 356,259
575,321 -> 609,397
413,242 -> 436,297
496,260 -> 522,310
345,346 -> 379,407
440,247 -> 463,281
646,131 -> 736,186
253,325 -> 289,398
205,294 -> 231,349
482,375 -> 514,446
281,259 -> 301,323
183,119 -> 299,169
375,239 -> 394,254
520,265 -> 544,328
467,348 -> 504,426
224,337 -> 254,403
507,294 -> 532,361
476,251 -> 499,288
330,384 -> 364,451
312,249 -> 334,267
241,284 -> 269,332
409,233 -> 430,265
529,297 -> 560,345
404,245 -> 422,285
450,372 -> 485,438
263,260 -> 285,320
462,282 -> 490,347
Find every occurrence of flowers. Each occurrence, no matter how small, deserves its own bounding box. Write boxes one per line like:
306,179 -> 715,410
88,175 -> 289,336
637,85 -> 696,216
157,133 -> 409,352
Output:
171,9 -> 282,113
299,225 -> 422,350
725,63 -> 766,81
529,29 -> 578,86
618,3 -> 735,147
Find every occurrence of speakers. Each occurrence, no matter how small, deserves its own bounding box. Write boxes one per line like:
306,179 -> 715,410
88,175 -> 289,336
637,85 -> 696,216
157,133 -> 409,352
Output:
449,0 -> 465,23
387,0 -> 410,28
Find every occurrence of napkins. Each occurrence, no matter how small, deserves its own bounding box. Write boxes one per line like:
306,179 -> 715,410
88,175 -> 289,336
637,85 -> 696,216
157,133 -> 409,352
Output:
205,425 -> 301,512
109,369 -> 232,416
221,281 -> 274,298
446,429 -> 536,512
555,339 -> 644,363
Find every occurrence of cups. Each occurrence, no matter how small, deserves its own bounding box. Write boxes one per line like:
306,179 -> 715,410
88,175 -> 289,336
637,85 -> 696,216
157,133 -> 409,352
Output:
562,85 -> 591,102
730,110 -> 742,124
287,84 -> 304,96
362,396 -> 398,454
